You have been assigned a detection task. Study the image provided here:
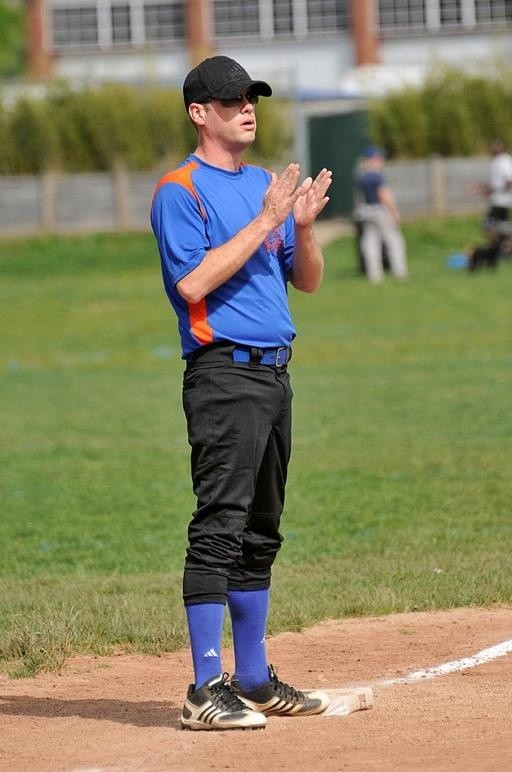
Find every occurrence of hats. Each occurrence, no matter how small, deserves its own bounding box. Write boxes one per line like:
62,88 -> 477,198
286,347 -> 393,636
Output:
183,55 -> 273,113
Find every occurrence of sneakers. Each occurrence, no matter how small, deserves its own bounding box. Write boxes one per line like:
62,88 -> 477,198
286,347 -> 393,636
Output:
181,664 -> 330,731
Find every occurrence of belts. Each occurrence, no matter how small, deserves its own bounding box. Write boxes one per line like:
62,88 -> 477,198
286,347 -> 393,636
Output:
231,345 -> 296,367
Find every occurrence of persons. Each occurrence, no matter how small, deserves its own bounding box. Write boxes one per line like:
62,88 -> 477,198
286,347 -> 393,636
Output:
481,140 -> 512,258
150,56 -> 333,730
353,143 -> 409,285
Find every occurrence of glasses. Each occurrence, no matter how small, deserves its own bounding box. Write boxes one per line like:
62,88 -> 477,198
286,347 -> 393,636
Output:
203,89 -> 259,107
